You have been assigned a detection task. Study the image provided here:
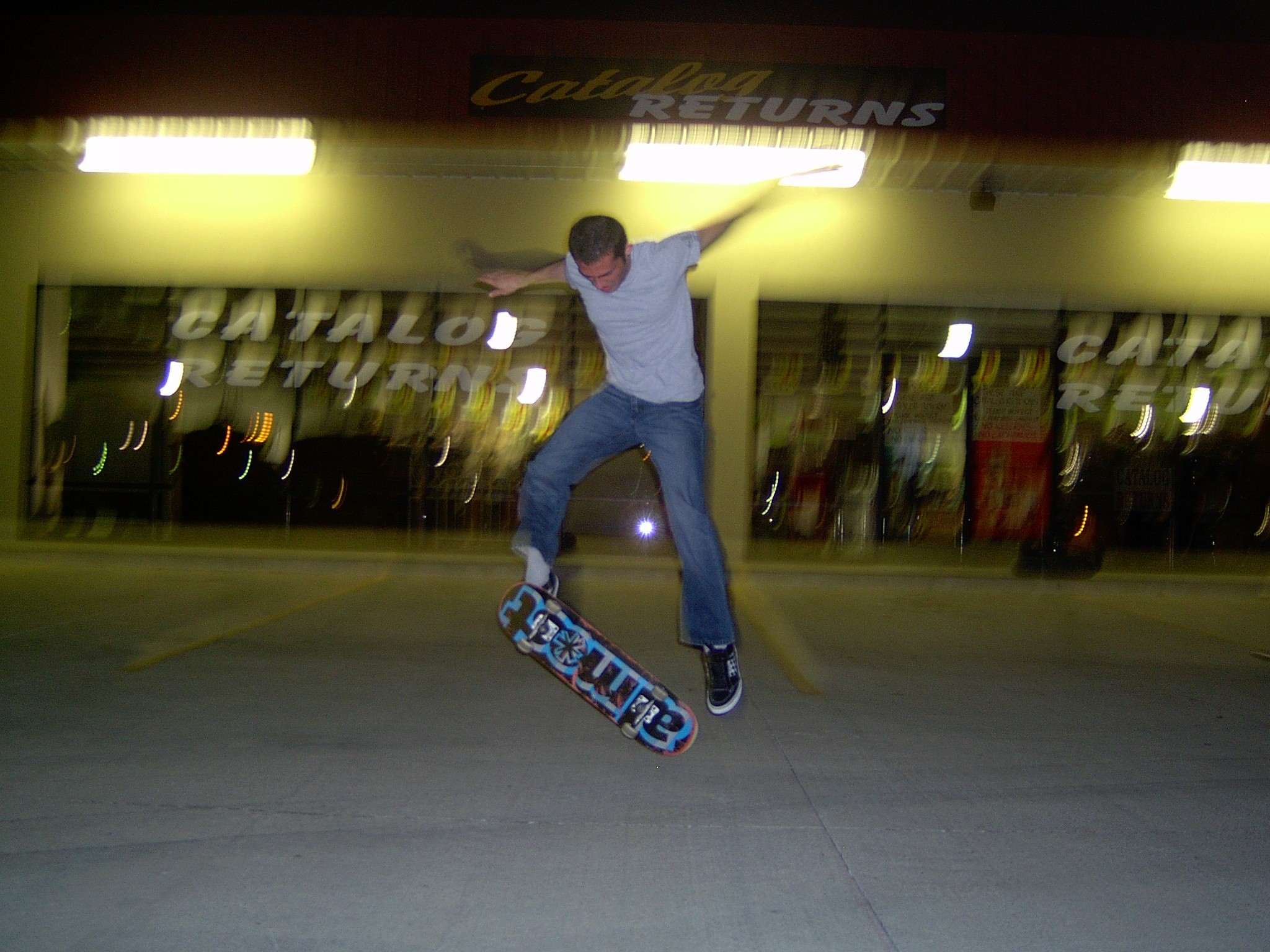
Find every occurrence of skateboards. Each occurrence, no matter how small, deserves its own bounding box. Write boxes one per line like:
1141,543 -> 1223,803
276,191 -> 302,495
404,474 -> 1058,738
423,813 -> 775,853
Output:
497,580 -> 700,756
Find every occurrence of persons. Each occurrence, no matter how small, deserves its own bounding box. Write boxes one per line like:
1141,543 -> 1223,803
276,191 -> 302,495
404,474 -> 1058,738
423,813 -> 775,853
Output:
474,180 -> 780,716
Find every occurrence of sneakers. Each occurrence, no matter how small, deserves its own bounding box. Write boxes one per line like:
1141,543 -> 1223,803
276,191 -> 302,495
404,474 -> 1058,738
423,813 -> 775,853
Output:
544,569 -> 559,596
703,642 -> 742,715
